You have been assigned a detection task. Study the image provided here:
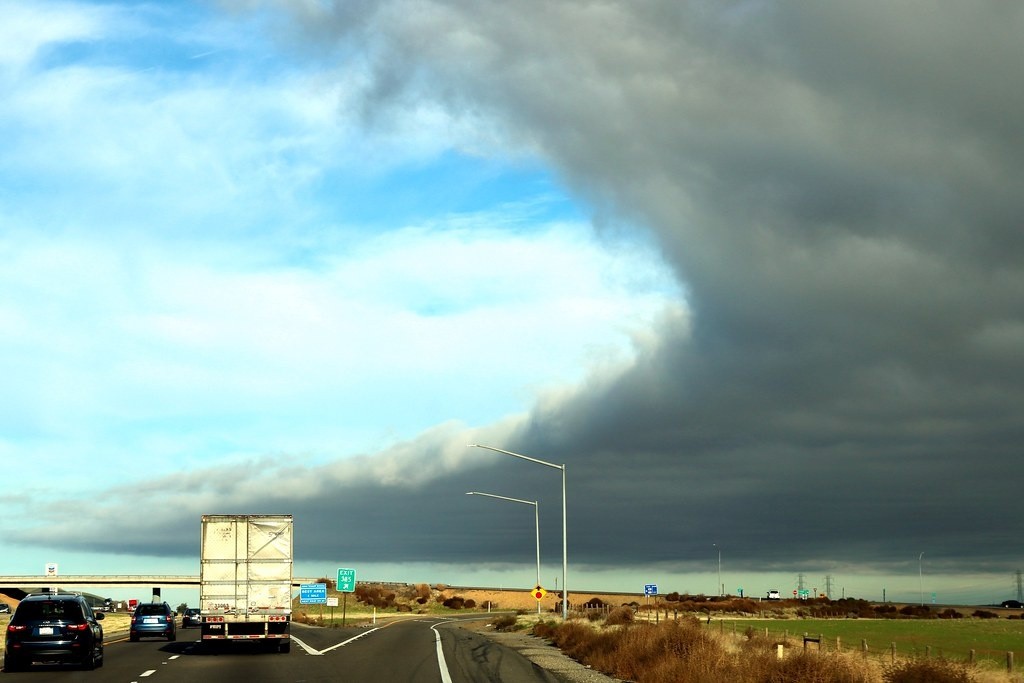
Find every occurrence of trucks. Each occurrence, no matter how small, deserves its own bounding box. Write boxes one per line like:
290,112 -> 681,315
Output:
199,514 -> 294,654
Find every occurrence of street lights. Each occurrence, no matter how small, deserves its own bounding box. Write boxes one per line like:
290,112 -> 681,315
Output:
467,491 -> 541,614
919,552 -> 924,606
713,543 -> 721,596
467,441 -> 568,621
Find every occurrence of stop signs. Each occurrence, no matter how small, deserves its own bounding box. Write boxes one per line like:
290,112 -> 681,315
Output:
793,590 -> 798,596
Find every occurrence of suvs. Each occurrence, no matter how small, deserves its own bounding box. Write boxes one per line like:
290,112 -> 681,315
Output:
130,601 -> 178,642
766,589 -> 780,600
4,592 -> 106,673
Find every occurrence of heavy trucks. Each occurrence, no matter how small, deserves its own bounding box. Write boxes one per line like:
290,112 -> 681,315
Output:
128,600 -> 139,612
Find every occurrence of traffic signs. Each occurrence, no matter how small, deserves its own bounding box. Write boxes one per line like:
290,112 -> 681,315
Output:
336,567 -> 356,593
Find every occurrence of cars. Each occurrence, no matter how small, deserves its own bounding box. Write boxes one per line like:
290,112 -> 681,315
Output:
182,608 -> 200,628
0,603 -> 11,614
1002,600 -> 1024,608
